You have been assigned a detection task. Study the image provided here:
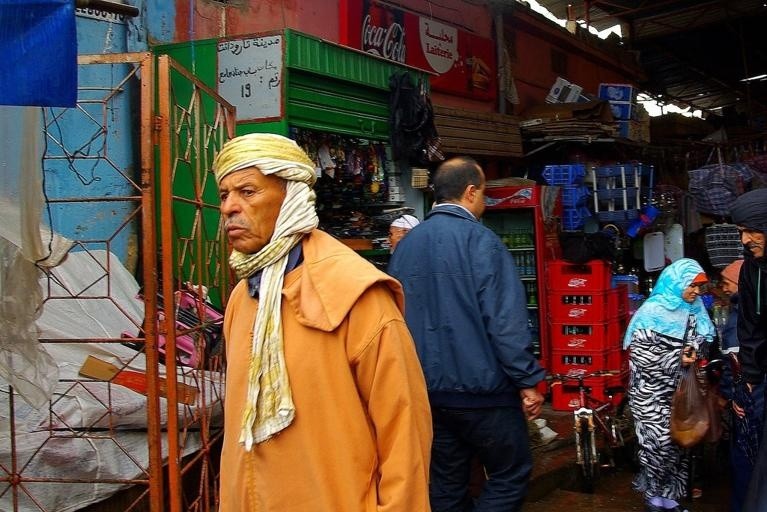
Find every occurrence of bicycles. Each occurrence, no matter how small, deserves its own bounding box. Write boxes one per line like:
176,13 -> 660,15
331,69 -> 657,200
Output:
541,364 -> 639,502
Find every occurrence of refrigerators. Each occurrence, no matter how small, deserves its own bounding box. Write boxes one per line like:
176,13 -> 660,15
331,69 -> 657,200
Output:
476,184 -> 566,402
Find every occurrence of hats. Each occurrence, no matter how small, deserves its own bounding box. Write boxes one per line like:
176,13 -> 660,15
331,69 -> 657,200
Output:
720,259 -> 744,285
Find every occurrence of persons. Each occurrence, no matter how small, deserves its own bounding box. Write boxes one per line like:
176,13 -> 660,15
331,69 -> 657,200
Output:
388,214 -> 419,254
212,132 -> 435,511
622,258 -> 716,510
717,188 -> 766,510
386,158 -> 549,512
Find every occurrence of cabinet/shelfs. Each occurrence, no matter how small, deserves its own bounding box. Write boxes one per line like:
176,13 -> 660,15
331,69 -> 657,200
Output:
145,28 -> 439,320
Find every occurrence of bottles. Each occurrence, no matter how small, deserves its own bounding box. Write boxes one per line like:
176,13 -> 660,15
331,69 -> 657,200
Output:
560,293 -> 593,366
483,216 -> 541,347
712,305 -> 729,332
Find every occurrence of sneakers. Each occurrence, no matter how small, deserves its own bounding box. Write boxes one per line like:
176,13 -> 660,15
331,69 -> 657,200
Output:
647,498 -> 684,512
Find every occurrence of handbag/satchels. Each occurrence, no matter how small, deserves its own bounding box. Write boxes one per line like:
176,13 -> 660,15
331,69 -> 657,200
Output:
688,146 -> 754,217
